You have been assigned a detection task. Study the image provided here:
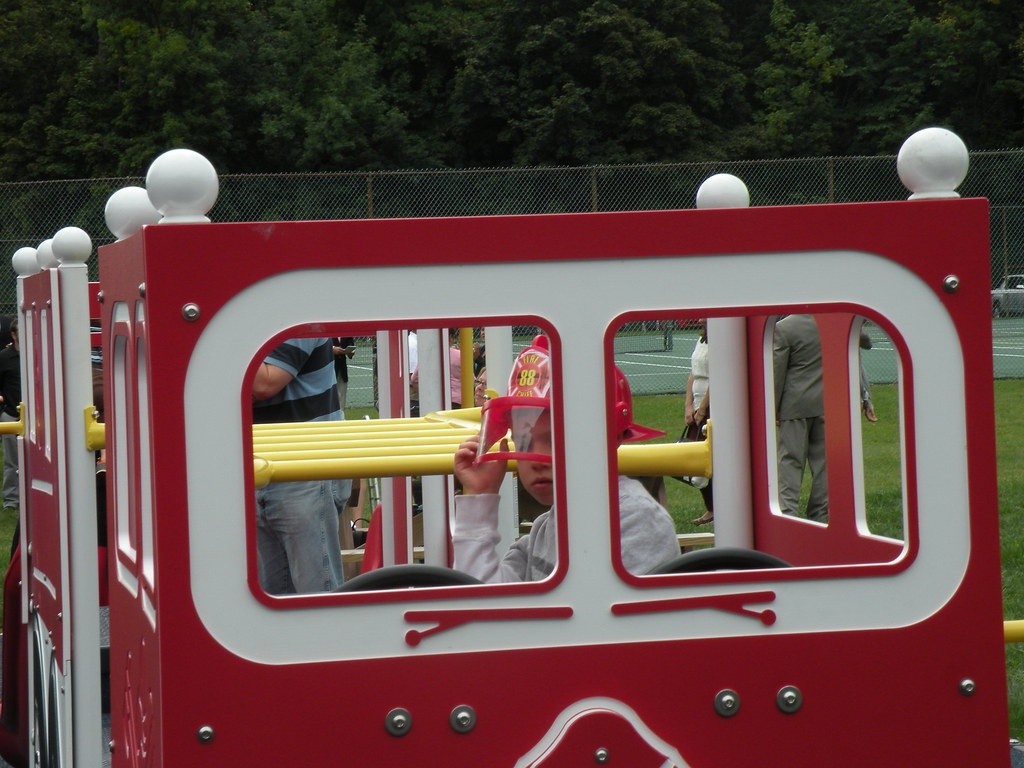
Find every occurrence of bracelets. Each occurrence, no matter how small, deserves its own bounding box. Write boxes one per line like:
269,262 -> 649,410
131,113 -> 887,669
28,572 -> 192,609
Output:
696,408 -> 706,416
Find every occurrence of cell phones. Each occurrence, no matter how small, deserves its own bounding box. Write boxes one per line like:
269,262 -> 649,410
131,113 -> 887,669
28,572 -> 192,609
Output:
344,345 -> 357,354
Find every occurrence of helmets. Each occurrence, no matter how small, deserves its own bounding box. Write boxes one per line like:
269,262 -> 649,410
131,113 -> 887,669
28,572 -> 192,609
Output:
473,335 -> 666,465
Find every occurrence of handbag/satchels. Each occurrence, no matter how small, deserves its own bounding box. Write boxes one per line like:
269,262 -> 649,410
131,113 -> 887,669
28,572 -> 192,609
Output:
670,418 -> 710,489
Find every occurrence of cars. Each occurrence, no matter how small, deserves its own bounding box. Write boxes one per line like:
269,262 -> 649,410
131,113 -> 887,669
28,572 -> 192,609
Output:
990,274 -> 1024,317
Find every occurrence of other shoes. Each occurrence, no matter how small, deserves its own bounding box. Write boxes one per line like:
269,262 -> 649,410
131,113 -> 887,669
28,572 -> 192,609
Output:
690,510 -> 714,525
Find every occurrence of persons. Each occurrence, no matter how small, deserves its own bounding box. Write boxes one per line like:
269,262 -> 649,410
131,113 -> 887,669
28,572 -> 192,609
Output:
0,317 -> 22,513
452,333 -> 683,588
407,329 -> 487,505
250,335 -> 366,597
685,312 -> 880,528
11,414 -> 113,768
